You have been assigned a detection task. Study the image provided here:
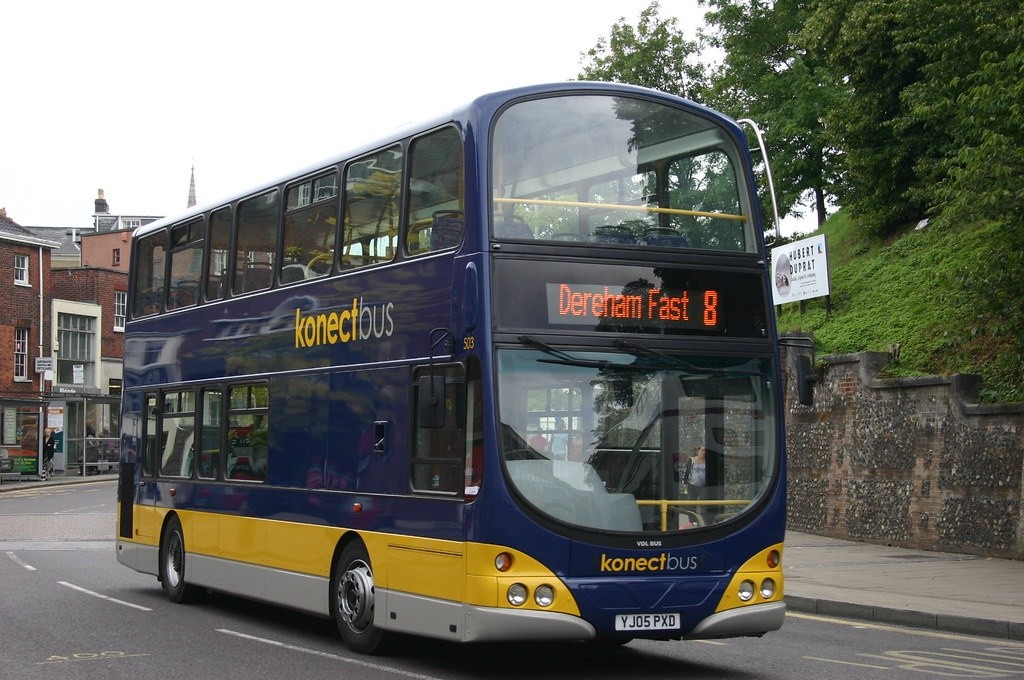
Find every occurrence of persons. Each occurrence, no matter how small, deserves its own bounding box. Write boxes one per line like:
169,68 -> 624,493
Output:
41,428 -> 56,481
78,419 -> 113,476
529,420 -> 584,461
465,444 -> 483,501
683,445 -> 706,526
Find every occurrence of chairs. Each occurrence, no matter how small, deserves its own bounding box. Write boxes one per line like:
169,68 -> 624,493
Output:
133,262 -> 274,319
430,211 -> 692,251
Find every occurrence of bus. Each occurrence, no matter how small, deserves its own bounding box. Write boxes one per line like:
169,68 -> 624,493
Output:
113,79 -> 820,657
